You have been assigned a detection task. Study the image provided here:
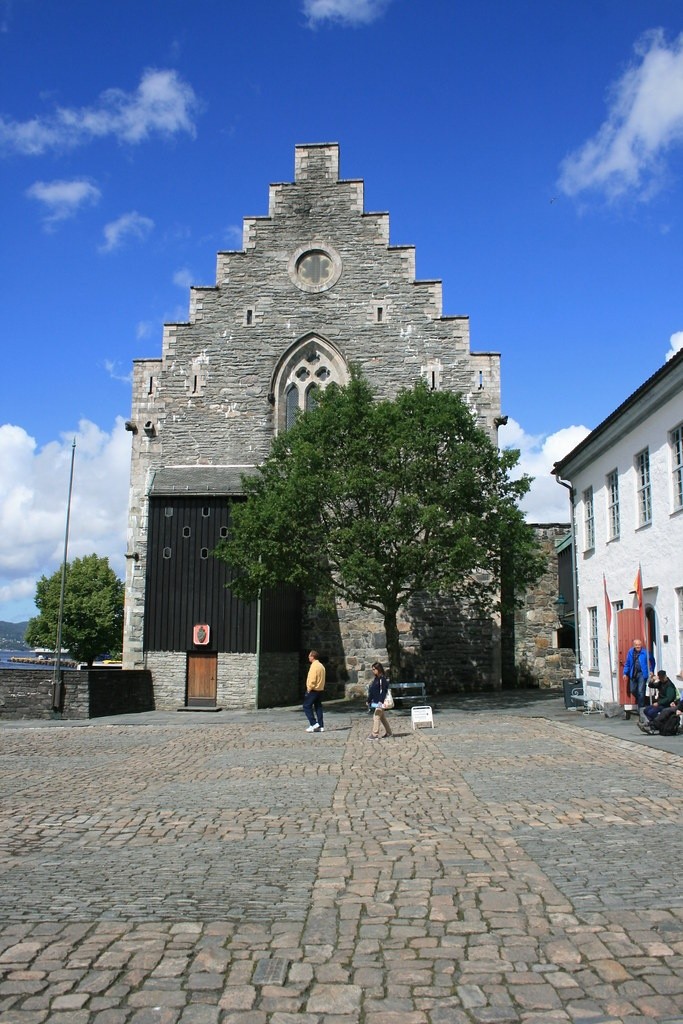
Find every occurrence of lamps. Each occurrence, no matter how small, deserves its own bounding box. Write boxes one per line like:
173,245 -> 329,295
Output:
553,592 -> 575,629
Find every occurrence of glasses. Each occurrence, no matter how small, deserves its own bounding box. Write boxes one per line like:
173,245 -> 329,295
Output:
372,669 -> 375,671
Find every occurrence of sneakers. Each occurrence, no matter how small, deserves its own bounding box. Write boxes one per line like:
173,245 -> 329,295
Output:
306,723 -> 320,732
315,727 -> 325,732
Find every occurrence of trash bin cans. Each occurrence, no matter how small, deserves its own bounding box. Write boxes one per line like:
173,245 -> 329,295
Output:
563,678 -> 584,709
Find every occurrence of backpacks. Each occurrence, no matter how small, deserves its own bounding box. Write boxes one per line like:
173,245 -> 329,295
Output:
659,714 -> 680,736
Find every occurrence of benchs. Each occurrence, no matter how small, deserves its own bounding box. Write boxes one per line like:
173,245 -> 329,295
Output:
567,680 -> 604,716
364,683 -> 428,714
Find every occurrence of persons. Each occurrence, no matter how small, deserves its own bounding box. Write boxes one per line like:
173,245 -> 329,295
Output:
366,662 -> 393,739
303,650 -> 325,733
637,670 -> 683,736
622,639 -> 655,708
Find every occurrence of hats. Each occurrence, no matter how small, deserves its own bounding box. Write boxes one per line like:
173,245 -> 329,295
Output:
657,670 -> 666,678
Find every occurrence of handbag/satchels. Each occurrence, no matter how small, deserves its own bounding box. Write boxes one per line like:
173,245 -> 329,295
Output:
380,688 -> 394,709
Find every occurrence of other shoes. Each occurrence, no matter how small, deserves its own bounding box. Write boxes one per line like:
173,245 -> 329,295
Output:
382,733 -> 393,739
367,734 -> 378,740
643,726 -> 655,734
637,722 -> 648,732
